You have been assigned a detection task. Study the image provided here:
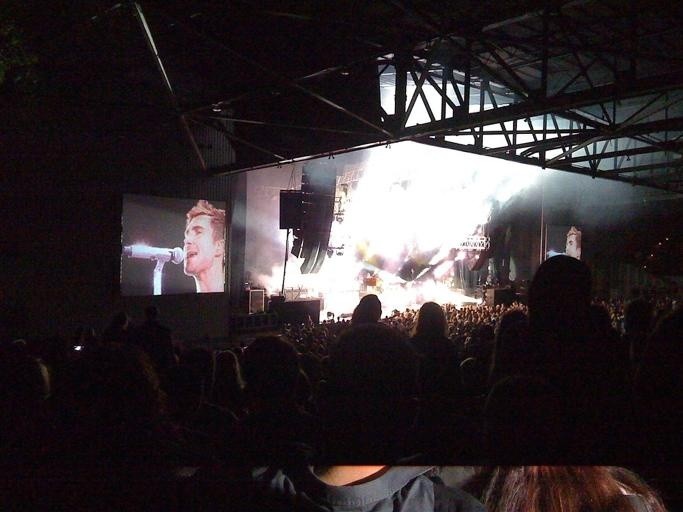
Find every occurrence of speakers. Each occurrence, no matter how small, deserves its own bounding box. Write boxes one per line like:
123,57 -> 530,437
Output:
292,160 -> 336,275
279,189 -> 305,229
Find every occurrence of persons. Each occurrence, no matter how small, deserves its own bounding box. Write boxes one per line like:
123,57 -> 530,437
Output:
181,201 -> 223,292
564,225 -> 579,258
1,255 -> 683,512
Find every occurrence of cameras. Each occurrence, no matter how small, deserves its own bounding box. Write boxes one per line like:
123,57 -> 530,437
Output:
74,345 -> 84,352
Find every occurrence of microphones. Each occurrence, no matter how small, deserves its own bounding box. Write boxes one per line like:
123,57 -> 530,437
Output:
122,244 -> 185,265
546,250 -> 566,259
